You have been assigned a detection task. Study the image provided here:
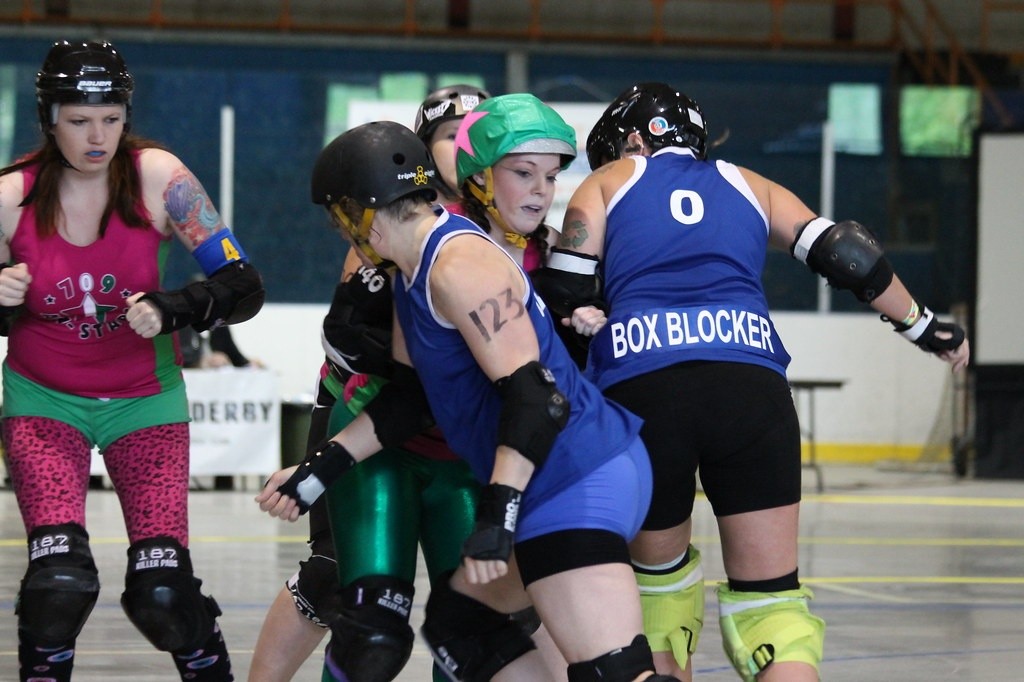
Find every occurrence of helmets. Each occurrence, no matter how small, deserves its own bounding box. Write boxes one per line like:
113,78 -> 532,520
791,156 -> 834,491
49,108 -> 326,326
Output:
414,84 -> 492,138
36,38 -> 134,134
585,82 -> 709,172
453,93 -> 578,191
311,121 -> 438,210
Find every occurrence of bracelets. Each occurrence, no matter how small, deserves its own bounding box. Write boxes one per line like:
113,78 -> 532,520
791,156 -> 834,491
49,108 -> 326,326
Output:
890,301 -> 920,325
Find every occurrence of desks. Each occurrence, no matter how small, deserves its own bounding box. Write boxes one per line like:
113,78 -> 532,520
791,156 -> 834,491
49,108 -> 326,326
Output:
89,367 -> 281,490
787,378 -> 844,491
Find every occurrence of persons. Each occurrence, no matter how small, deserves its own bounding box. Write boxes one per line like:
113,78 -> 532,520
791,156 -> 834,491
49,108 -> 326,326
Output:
173,273 -> 269,489
320,93 -> 577,681
541,82 -> 969,682
1,36 -> 264,682
254,121 -> 686,682
248,84 -> 493,682
